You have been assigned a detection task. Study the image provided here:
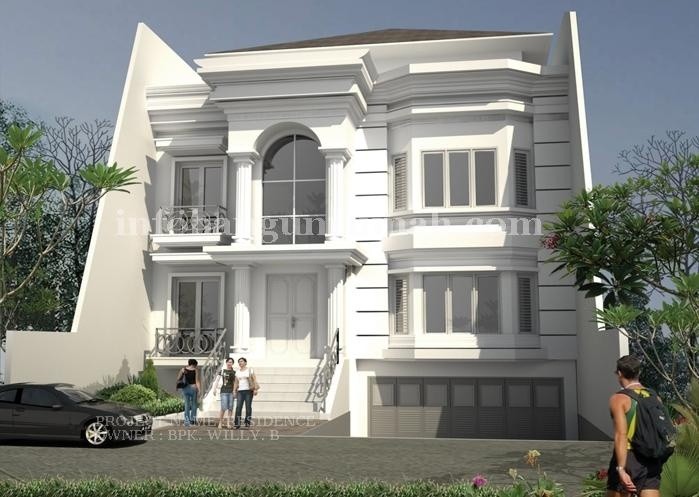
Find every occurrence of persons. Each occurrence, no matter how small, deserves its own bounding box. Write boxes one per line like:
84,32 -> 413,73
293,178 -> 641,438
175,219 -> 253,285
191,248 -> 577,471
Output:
604,354 -> 664,496
213,357 -> 236,428
233,357 -> 257,428
176,358 -> 201,426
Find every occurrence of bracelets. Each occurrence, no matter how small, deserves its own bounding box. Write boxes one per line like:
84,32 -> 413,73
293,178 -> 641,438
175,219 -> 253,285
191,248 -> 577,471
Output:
615,464 -> 624,471
